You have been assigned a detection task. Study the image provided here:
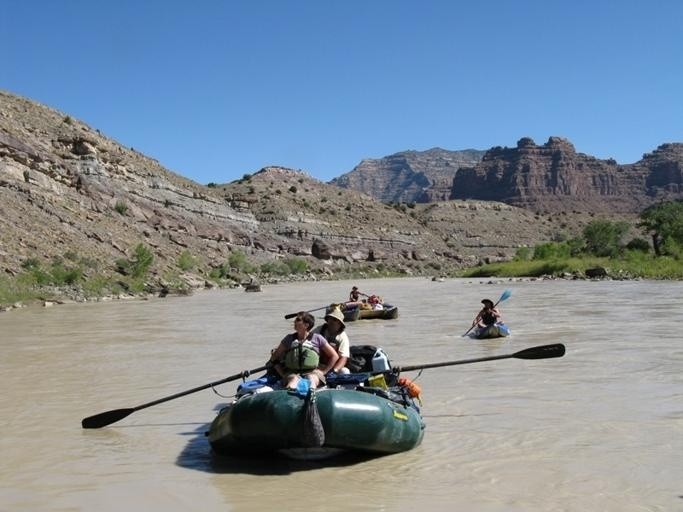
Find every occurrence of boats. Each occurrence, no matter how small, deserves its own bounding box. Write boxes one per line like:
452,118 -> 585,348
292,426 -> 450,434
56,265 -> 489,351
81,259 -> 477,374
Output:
207,350 -> 426,457
327,299 -> 400,321
473,323 -> 510,338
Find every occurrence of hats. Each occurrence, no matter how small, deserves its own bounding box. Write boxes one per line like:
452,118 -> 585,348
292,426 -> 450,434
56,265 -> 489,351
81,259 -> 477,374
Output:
323,308 -> 346,330
480,298 -> 494,306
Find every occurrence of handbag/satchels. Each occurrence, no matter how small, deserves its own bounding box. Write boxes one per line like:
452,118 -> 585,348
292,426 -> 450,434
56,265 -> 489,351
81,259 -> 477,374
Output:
369,347 -> 391,372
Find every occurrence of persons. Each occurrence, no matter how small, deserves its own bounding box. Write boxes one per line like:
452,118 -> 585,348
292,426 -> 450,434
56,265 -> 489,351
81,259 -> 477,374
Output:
472,299 -> 504,328
310,308 -> 351,375
350,286 -> 364,302
270,311 -> 339,389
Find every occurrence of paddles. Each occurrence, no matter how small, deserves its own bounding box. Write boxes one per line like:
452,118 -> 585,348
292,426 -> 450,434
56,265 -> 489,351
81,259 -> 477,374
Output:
465,289 -> 512,336
285,305 -> 326,319
362,343 -> 565,373
82,366 -> 266,429
